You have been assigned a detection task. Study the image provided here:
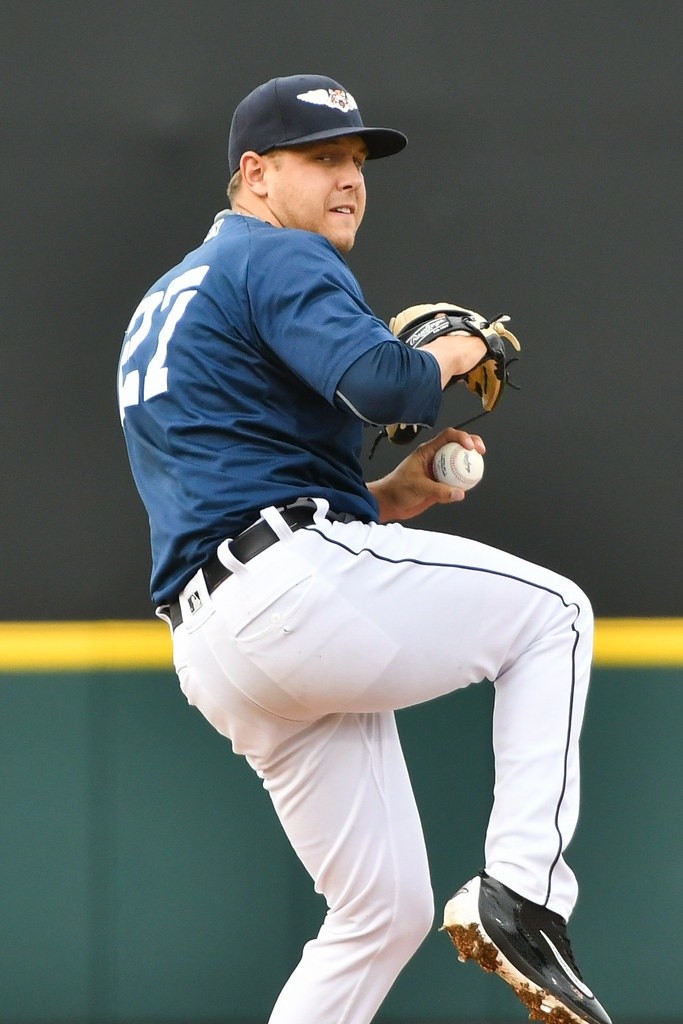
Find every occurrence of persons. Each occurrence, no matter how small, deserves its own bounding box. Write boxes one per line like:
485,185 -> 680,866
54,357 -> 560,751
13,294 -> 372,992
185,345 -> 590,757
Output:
117,74 -> 612,1024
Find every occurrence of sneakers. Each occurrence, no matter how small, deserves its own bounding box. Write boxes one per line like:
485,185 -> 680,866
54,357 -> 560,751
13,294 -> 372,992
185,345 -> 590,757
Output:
444,870 -> 611,1024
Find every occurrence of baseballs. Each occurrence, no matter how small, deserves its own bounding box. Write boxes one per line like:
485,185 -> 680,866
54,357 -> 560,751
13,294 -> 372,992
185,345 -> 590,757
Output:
432,442 -> 485,491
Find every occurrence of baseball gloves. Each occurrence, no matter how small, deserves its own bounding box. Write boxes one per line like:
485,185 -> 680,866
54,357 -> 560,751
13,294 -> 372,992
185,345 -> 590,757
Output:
384,302 -> 507,445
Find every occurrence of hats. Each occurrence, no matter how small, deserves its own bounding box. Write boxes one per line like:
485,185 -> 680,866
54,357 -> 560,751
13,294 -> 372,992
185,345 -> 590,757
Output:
229,75 -> 407,175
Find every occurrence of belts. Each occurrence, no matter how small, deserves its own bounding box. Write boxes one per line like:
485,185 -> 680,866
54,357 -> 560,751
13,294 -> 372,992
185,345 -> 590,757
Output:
167,505 -> 334,631
